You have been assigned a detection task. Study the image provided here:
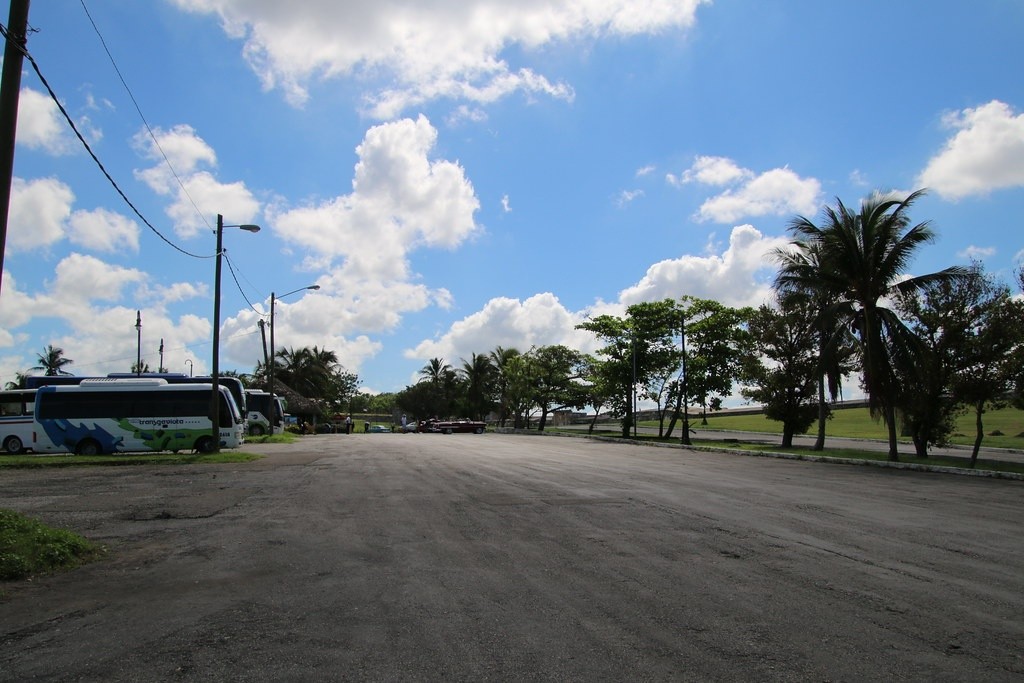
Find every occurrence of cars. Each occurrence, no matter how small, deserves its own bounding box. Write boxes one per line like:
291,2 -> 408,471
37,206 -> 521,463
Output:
366,425 -> 391,432
399,417 -> 443,433
245,388 -> 286,437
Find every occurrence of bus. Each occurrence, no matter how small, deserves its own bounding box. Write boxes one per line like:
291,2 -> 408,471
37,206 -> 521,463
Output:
0,388 -> 38,454
32,376 -> 246,454
107,371 -> 249,433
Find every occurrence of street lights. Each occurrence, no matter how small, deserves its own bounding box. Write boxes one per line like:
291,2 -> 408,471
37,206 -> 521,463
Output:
206,213 -> 261,454
135,309 -> 143,377
268,285 -> 321,432
185,359 -> 193,377
159,337 -> 165,373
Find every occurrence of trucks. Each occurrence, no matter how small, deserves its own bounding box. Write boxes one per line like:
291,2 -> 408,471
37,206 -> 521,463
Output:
434,417 -> 487,434
